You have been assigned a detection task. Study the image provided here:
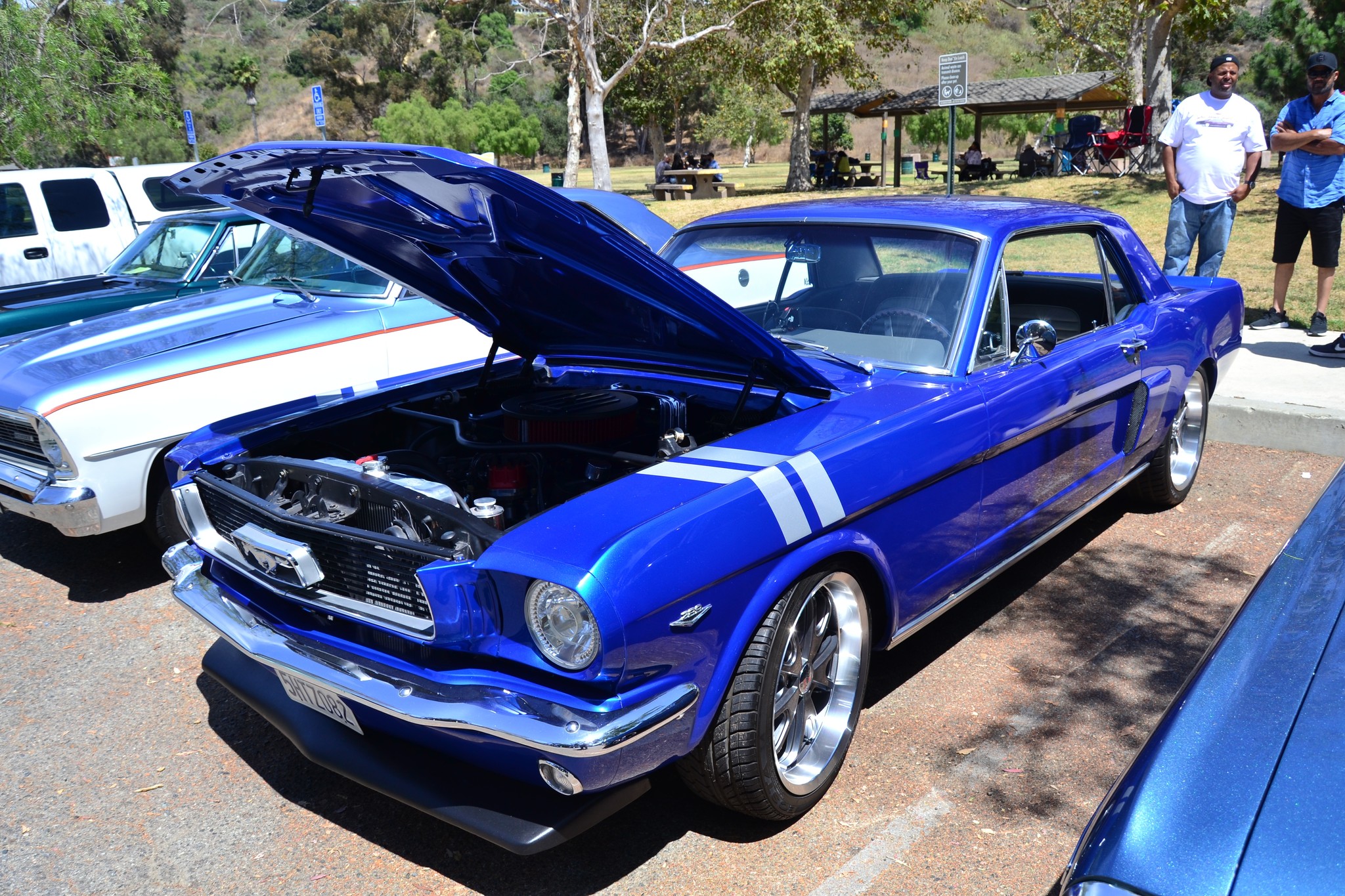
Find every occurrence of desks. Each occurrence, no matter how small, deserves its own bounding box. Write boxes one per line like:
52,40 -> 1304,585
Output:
941,160 -> 1004,180
664,168 -> 730,199
819,161 -> 881,173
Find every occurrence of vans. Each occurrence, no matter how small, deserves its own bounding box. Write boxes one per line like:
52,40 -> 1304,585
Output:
0,162 -> 299,304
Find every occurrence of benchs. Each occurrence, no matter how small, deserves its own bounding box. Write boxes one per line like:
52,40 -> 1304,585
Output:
863,272 -> 1120,334
643,182 -> 745,200
835,171 -> 883,186
931,170 -> 1016,182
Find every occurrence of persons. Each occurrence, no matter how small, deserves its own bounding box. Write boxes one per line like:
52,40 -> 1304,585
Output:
959,142 -> 982,182
810,149 -> 850,189
1019,145 -> 1048,178
1249,51 -> 1345,337
1157,54 -> 1268,278
656,151 -> 723,194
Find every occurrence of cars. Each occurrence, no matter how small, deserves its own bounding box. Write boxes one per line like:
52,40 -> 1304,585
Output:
0,187 -> 812,539
1046,460 -> 1345,896
141,140 -> 1244,854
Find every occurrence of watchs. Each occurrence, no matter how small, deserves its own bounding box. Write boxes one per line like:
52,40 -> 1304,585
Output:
1244,180 -> 1255,189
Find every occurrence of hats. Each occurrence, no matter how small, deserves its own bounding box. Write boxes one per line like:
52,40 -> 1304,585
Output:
1206,54 -> 1239,87
706,152 -> 714,157
1307,51 -> 1337,70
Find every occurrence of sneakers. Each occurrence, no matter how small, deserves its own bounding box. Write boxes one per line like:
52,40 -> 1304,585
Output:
1250,308 -> 1288,330
1308,312 -> 1327,336
1309,333 -> 1345,358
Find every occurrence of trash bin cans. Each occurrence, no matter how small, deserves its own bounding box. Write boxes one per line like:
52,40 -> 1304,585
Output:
551,173 -> 563,186
901,157 -> 913,174
933,153 -> 939,161
543,164 -> 549,172
865,154 -> 870,160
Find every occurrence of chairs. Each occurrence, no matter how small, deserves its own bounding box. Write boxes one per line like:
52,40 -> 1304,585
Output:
874,296 -> 947,339
809,151 -> 839,189
914,160 -> 939,185
1007,303 -> 1081,346
1044,104 -> 1156,177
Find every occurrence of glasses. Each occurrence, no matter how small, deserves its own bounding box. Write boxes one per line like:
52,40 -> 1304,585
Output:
1308,69 -> 1333,79
